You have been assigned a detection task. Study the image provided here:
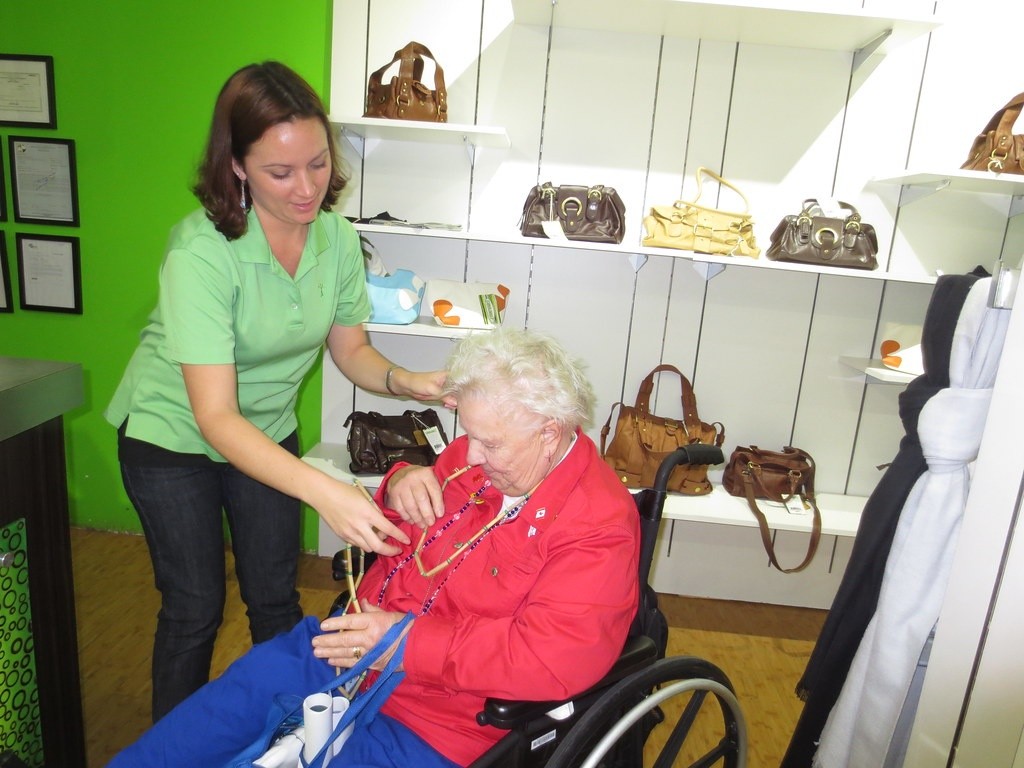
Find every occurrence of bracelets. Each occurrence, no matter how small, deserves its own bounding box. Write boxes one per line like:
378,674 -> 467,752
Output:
386,364 -> 403,395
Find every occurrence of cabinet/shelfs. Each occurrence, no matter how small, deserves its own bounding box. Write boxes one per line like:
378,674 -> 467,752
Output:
304,0 -> 1024,538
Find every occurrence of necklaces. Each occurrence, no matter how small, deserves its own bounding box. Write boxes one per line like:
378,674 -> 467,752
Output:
413,465 -> 556,577
336,481 -> 385,700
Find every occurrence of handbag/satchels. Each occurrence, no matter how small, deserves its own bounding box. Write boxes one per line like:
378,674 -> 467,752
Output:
358,234 -> 425,324
722,444 -> 810,498
343,408 -> 449,478
958,92 -> 1024,175
641,168 -> 760,257
767,194 -> 880,269
365,42 -> 448,124
522,184 -> 626,244
597,366 -> 727,497
428,279 -> 510,330
221,608 -> 416,768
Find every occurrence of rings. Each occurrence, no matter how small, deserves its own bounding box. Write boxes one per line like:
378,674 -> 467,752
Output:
353,646 -> 361,660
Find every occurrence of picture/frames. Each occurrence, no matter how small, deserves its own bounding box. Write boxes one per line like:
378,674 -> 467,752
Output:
15,232 -> 83,315
0,53 -> 58,130
0,230 -> 15,314
6,134 -> 82,228
0,135 -> 9,222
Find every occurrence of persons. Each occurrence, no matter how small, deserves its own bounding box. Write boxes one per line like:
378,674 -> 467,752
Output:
117,61 -> 462,730
108,325 -> 642,768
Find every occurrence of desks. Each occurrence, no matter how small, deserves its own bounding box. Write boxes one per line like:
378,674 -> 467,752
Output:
0,353 -> 87,768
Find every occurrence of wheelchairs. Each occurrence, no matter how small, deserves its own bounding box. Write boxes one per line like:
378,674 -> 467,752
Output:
329,445 -> 749,768
